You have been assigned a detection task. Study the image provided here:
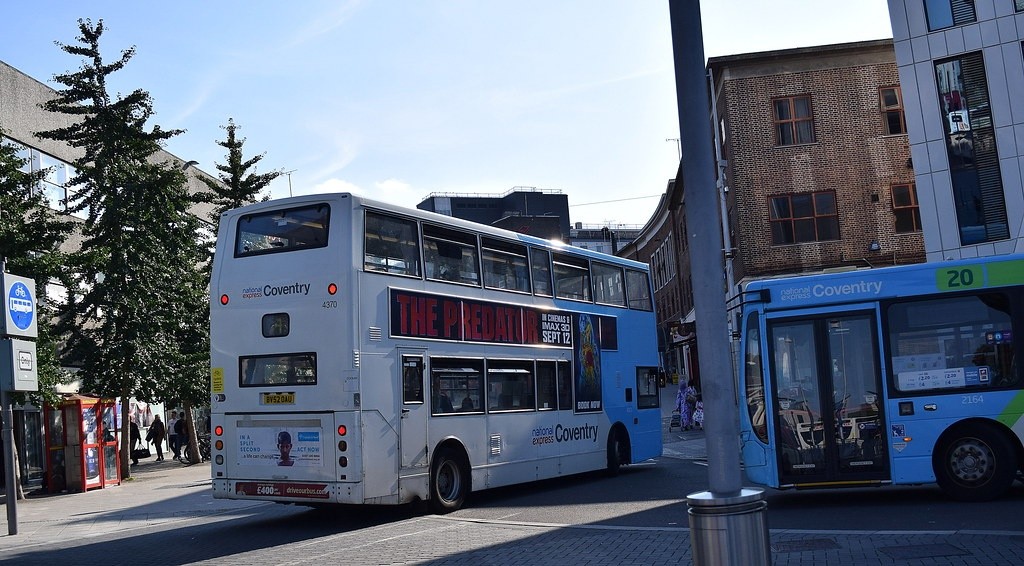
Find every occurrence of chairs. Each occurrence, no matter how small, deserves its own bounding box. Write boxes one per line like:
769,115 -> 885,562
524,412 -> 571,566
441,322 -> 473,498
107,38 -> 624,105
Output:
437,390 -> 574,414
442,268 -> 472,285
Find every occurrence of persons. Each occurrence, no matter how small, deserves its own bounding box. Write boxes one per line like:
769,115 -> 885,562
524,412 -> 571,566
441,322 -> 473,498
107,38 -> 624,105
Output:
103,421 -> 110,442
130,416 -> 141,467
173,412 -> 189,459
146,414 -> 166,461
197,408 -> 211,433
676,380 -> 704,431
168,411 -> 181,459
274,431 -> 301,466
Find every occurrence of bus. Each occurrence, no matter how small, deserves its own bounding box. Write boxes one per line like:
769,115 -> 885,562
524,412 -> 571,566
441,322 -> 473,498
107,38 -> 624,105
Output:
211,192 -> 664,514
737,252 -> 1024,504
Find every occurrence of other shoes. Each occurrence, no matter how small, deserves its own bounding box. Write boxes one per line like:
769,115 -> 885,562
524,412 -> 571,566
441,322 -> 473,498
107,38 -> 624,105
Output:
178,456 -> 181,460
130,462 -> 137,467
162,458 -> 164,460
173,457 -> 178,461
156,458 -> 161,461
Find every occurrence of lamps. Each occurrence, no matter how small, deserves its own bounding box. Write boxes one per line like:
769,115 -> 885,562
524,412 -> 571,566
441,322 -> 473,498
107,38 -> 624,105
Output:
869,240 -> 880,251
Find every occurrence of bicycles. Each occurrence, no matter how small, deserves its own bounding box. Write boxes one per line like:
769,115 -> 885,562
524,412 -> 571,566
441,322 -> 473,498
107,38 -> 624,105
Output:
177,429 -> 211,465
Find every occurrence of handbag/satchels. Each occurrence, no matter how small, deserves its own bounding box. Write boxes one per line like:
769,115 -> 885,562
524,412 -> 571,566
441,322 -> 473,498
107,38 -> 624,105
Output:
130,444 -> 151,459
